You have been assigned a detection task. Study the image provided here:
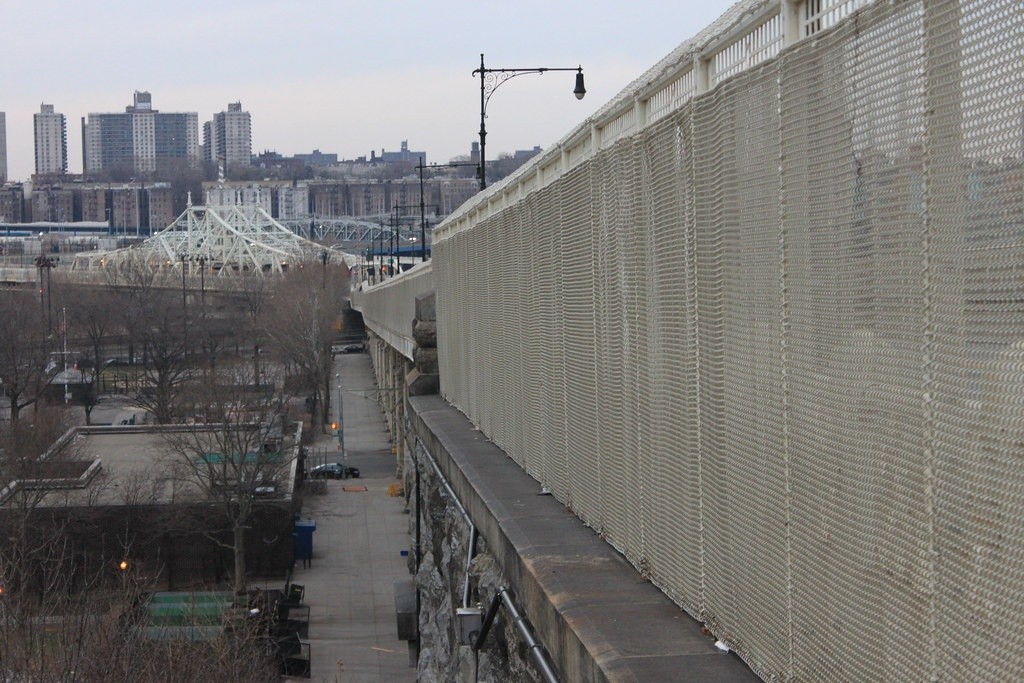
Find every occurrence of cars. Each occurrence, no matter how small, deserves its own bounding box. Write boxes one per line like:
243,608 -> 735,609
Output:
330,346 -> 348,354
344,345 -> 365,353
311,463 -> 360,478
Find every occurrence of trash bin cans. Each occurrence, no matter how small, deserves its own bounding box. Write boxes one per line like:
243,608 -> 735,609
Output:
294,520 -> 316,559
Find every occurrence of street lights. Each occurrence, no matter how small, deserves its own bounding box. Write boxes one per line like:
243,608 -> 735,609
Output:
371,233 -> 392,286
119,562 -> 128,599
105,207 -> 112,252
472,50 -> 587,189
393,200 -> 442,273
379,220 -> 413,282
414,156 -> 481,265
389,214 -> 430,277
0,588 -> 8,646
